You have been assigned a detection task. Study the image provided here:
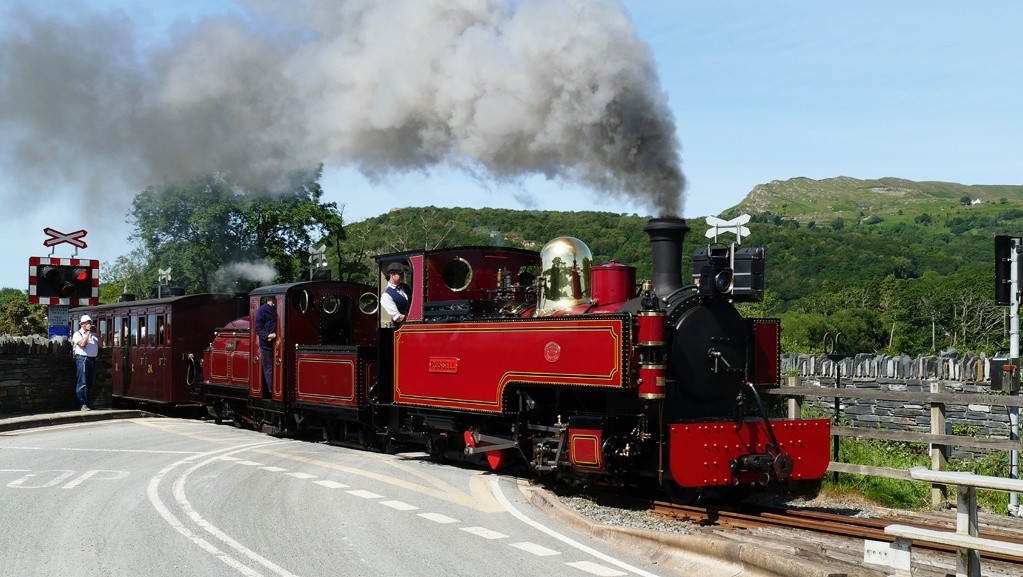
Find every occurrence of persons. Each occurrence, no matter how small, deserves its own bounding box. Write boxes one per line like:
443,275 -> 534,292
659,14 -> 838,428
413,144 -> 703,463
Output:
254,296 -> 278,399
515,272 -> 537,301
72,315 -> 103,411
380,262 -> 413,327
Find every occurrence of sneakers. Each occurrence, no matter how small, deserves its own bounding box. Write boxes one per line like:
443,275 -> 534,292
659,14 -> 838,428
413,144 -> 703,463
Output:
81,404 -> 91,412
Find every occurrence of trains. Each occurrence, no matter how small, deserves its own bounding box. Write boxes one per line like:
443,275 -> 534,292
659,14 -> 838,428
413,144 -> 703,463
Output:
72,218 -> 833,506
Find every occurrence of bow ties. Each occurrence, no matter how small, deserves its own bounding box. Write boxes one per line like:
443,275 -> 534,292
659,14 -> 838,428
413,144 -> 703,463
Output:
396,284 -> 402,290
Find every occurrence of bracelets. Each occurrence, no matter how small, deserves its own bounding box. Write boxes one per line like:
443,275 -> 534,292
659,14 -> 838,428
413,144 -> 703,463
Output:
96,336 -> 101,338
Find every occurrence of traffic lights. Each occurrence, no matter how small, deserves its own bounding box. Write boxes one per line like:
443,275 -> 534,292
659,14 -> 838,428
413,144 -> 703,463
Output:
36,264 -> 92,299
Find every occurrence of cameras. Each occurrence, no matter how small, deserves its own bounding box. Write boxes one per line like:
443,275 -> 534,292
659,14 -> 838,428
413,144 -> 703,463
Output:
89,326 -> 96,332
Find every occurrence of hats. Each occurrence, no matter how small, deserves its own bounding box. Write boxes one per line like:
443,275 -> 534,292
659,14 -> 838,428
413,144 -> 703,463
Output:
387,262 -> 408,272
79,315 -> 93,324
